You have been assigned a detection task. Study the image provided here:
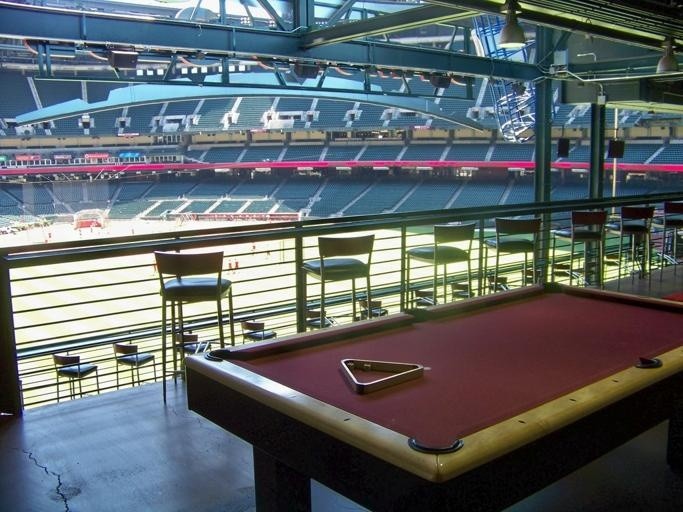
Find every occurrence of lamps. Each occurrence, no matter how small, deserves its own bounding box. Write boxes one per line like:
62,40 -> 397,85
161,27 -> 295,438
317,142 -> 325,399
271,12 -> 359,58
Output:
656,39 -> 679,74
497,1 -> 526,46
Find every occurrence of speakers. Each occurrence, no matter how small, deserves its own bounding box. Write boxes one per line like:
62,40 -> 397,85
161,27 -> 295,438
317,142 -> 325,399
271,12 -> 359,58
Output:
558,138 -> 569,157
110,51 -> 139,68
430,76 -> 451,87
294,64 -> 319,78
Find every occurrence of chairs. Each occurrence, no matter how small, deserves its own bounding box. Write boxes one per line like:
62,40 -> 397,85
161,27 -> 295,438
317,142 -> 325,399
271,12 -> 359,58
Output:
53,343 -> 156,401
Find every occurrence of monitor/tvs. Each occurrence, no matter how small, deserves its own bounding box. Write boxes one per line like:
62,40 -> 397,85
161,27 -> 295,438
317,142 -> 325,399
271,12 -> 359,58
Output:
608,141 -> 624,158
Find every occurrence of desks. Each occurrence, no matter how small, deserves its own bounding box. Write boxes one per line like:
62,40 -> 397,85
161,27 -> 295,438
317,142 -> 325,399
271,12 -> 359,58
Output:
185,281 -> 682,510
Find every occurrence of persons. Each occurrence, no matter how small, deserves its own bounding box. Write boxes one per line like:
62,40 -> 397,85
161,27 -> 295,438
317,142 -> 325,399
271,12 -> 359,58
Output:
250,241 -> 257,254
226,258 -> 234,277
232,257 -> 239,274
